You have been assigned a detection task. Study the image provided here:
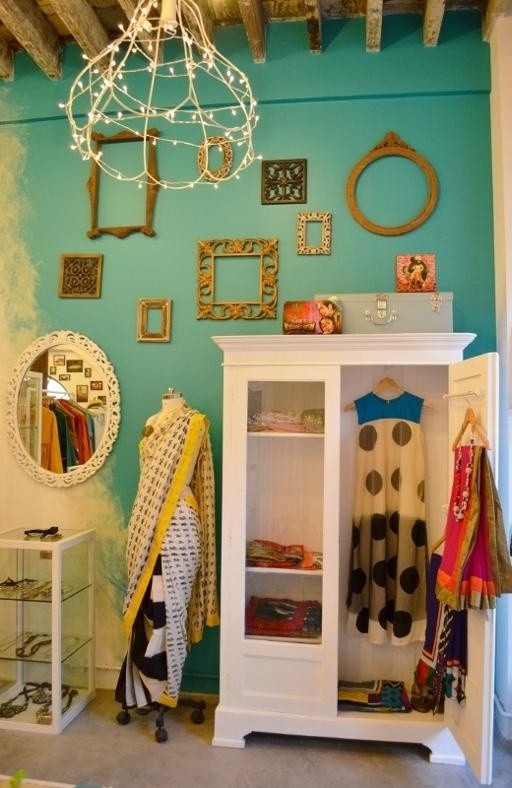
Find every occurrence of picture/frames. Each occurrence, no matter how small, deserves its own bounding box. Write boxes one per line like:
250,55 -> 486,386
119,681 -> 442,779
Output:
58,254 -> 103,298
49,355 -> 107,405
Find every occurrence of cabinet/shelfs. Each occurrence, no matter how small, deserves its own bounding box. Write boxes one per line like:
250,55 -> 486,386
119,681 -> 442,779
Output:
211,333 -> 499,784
0,527 -> 96,736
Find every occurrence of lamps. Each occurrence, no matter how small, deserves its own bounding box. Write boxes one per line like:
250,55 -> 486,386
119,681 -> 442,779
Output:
58,0 -> 262,191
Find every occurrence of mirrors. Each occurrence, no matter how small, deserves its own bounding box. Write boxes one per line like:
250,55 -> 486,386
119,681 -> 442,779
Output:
6,330 -> 121,488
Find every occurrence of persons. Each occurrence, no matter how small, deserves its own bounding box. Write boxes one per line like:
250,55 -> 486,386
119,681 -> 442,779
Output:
318,319 -> 336,334
114,394 -> 220,711
317,303 -> 341,331
407,257 -> 429,291
